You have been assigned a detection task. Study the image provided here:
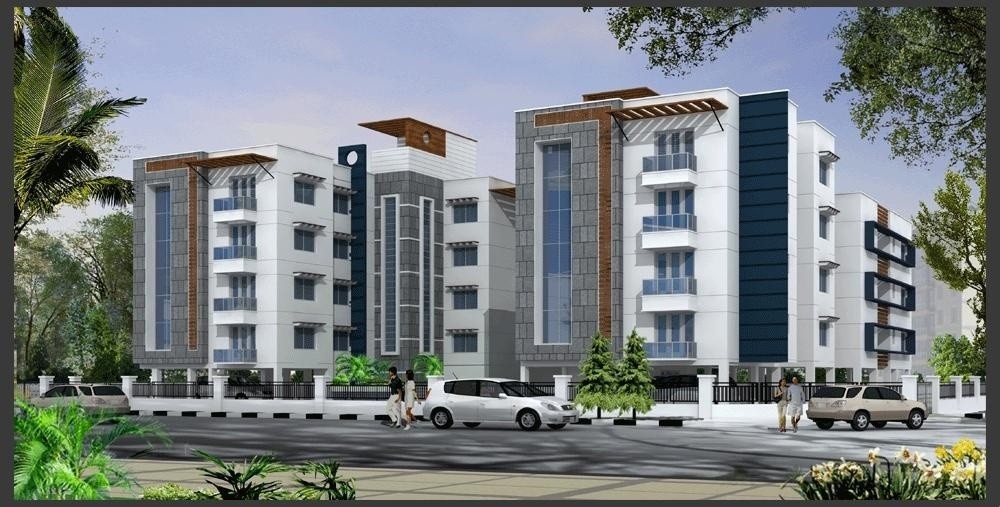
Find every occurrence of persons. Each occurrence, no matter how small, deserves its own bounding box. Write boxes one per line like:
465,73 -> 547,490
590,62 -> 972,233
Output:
787,377 -> 805,432
386,367 -> 402,428
402,370 -> 421,431
774,378 -> 788,433
